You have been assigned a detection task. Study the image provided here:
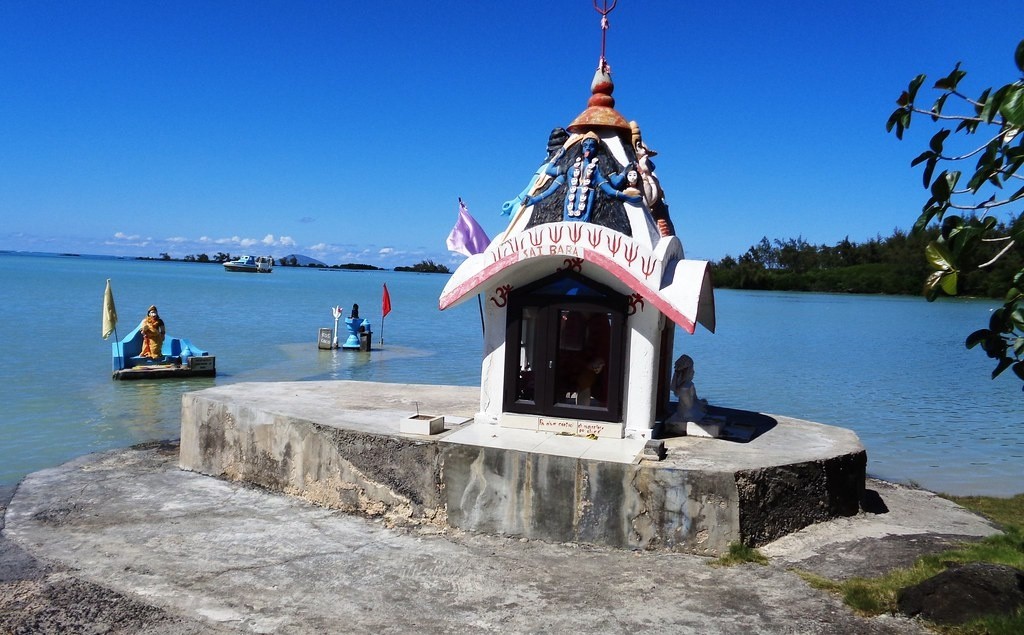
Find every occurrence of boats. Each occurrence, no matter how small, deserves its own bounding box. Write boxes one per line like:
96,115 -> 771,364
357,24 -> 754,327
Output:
112,320 -> 216,379
222,255 -> 277,273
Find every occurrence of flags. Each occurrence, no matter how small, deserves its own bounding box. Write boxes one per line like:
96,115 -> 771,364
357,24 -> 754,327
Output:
382,285 -> 392,318
102,279 -> 118,341
445,208 -> 491,257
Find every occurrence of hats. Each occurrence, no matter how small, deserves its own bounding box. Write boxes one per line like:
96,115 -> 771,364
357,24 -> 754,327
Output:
581,131 -> 601,144
674,354 -> 694,368
147,305 -> 156,311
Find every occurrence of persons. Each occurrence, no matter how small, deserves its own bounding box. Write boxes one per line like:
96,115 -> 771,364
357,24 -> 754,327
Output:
139,305 -> 166,361
